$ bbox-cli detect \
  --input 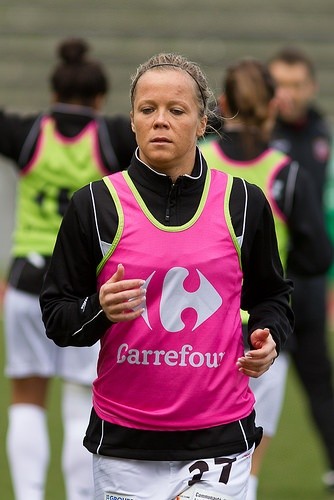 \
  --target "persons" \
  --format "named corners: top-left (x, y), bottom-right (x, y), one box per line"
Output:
top-left (0, 37), bottom-right (224, 500)
top-left (266, 48), bottom-right (333, 488)
top-left (194, 57), bottom-right (333, 499)
top-left (38, 54), bottom-right (298, 500)
